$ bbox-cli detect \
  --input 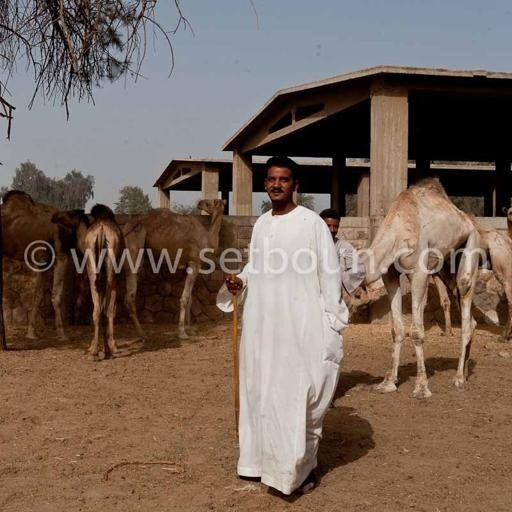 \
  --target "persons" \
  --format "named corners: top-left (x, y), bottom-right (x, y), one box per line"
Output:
top-left (319, 209), bottom-right (367, 302)
top-left (225, 156), bottom-right (349, 495)
